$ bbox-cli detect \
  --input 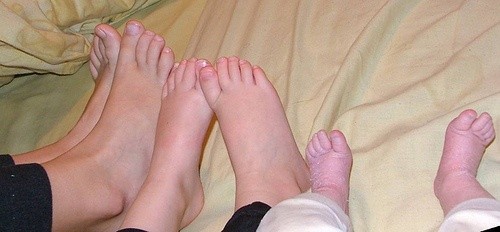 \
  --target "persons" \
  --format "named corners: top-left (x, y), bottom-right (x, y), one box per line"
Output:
top-left (116, 56), bottom-right (313, 232)
top-left (0, 20), bottom-right (175, 232)
top-left (256, 109), bottom-right (500, 232)
